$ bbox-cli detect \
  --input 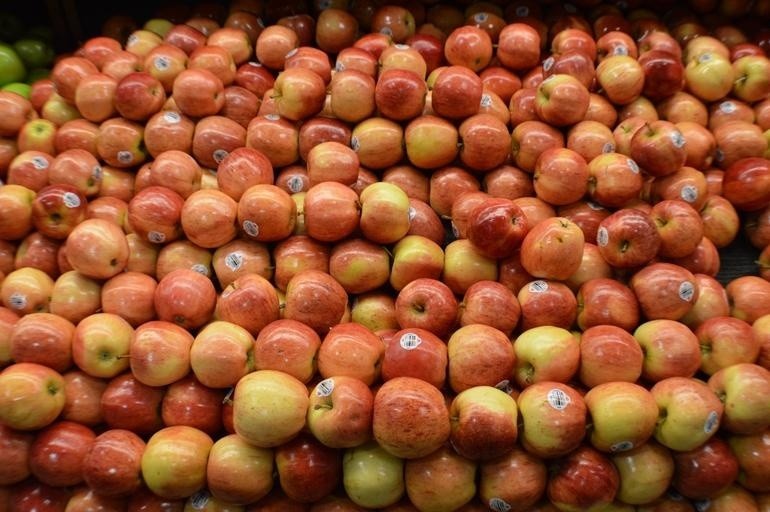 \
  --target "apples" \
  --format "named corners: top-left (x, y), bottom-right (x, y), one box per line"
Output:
top-left (0, 0), bottom-right (770, 512)
